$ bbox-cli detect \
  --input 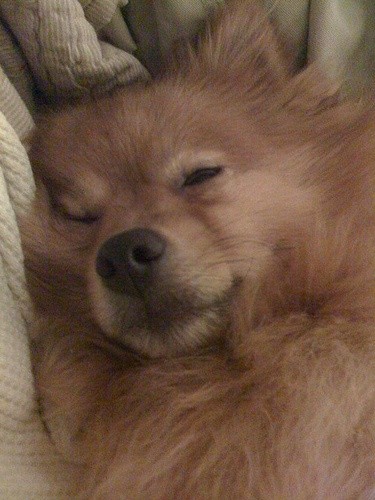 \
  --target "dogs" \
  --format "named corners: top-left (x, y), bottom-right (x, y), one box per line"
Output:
top-left (18, 1), bottom-right (375, 500)
